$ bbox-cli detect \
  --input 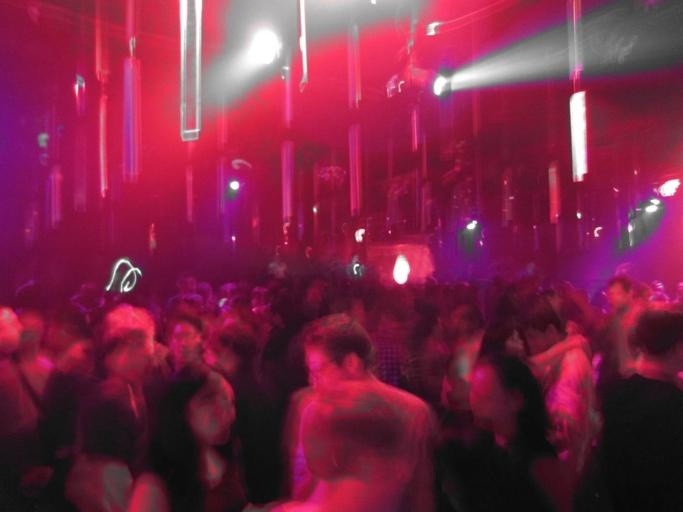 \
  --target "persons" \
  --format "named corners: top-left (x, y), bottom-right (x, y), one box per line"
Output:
top-left (0, 241), bottom-right (682, 511)
